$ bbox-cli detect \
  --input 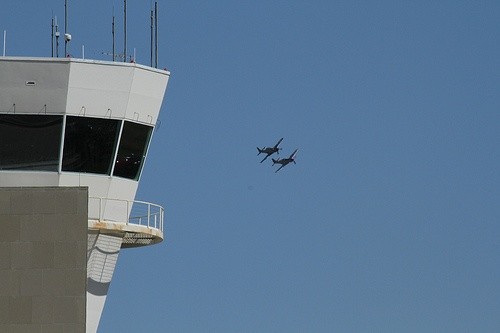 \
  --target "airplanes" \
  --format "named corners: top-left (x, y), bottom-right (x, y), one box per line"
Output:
top-left (272, 149), bottom-right (298, 173)
top-left (256, 138), bottom-right (284, 164)
